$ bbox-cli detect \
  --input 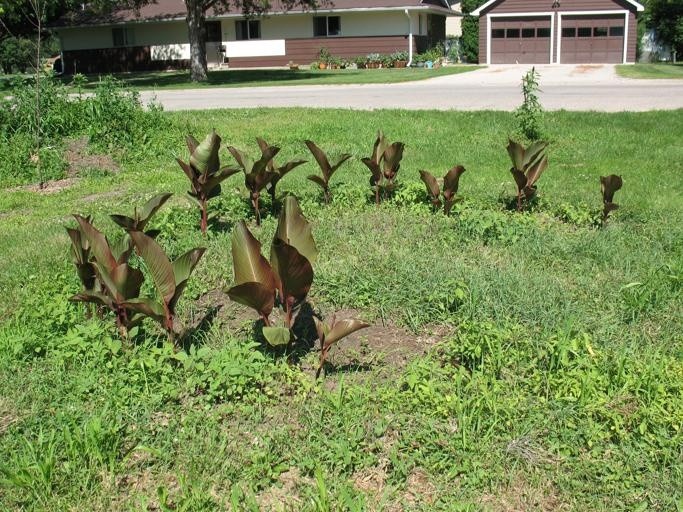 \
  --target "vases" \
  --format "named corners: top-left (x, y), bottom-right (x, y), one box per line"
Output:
top-left (307, 41), bottom-right (472, 70)
